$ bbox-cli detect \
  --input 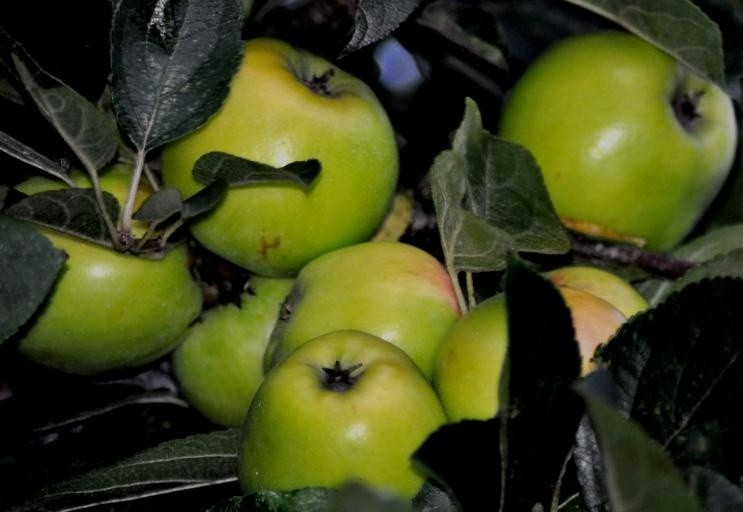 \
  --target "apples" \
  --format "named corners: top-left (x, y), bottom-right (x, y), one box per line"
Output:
top-left (541, 266), bottom-right (649, 320)
top-left (239, 328), bottom-right (448, 501)
top-left (161, 38), bottom-right (399, 280)
top-left (432, 285), bottom-right (627, 423)
top-left (277, 241), bottom-right (462, 385)
top-left (14, 165), bottom-right (203, 373)
top-left (499, 30), bottom-right (736, 256)
top-left (172, 276), bottom-right (294, 427)
